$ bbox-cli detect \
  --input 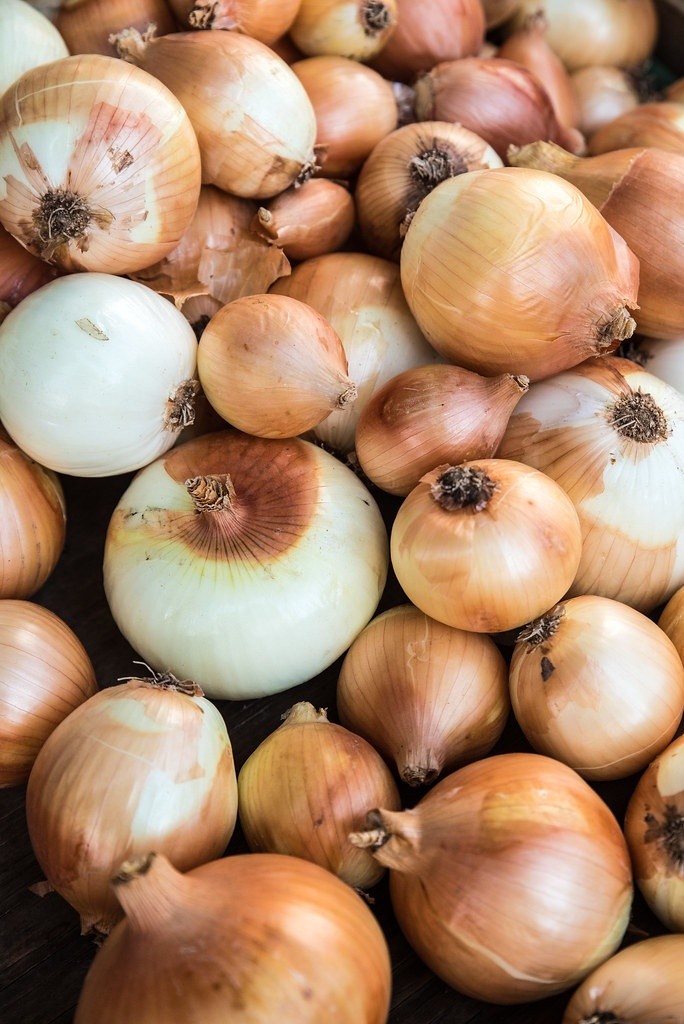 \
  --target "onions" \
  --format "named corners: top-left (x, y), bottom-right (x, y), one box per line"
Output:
top-left (0, 0), bottom-right (684, 1024)
